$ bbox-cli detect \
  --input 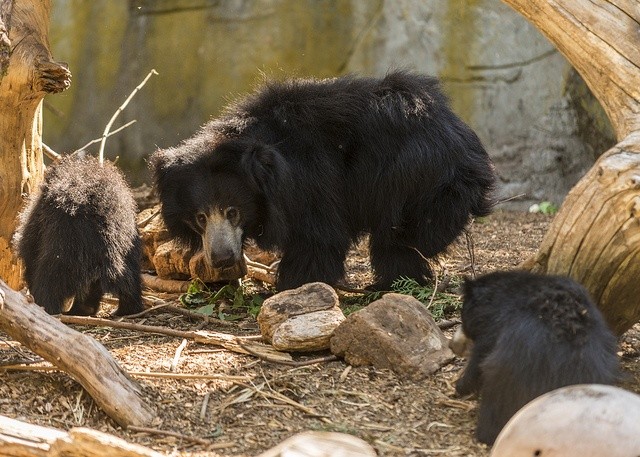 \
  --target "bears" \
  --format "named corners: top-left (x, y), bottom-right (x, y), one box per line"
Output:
top-left (9, 151), bottom-right (145, 326)
top-left (150, 69), bottom-right (496, 298)
top-left (455, 270), bottom-right (624, 446)
